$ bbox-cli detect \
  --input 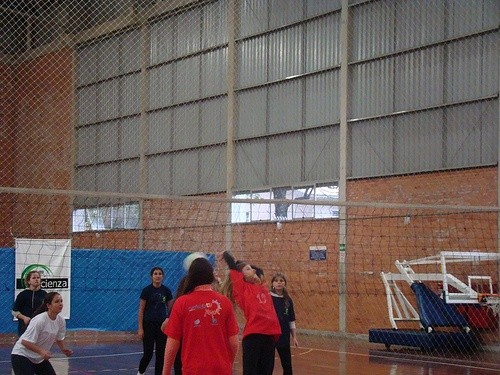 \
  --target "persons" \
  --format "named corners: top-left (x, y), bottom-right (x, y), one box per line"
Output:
top-left (267, 273), bottom-right (299, 375)
top-left (169, 274), bottom-right (193, 375)
top-left (10, 292), bottom-right (73, 375)
top-left (216, 250), bottom-right (282, 375)
top-left (11, 272), bottom-right (49, 338)
top-left (137, 266), bottom-right (174, 375)
top-left (161, 256), bottom-right (239, 375)
